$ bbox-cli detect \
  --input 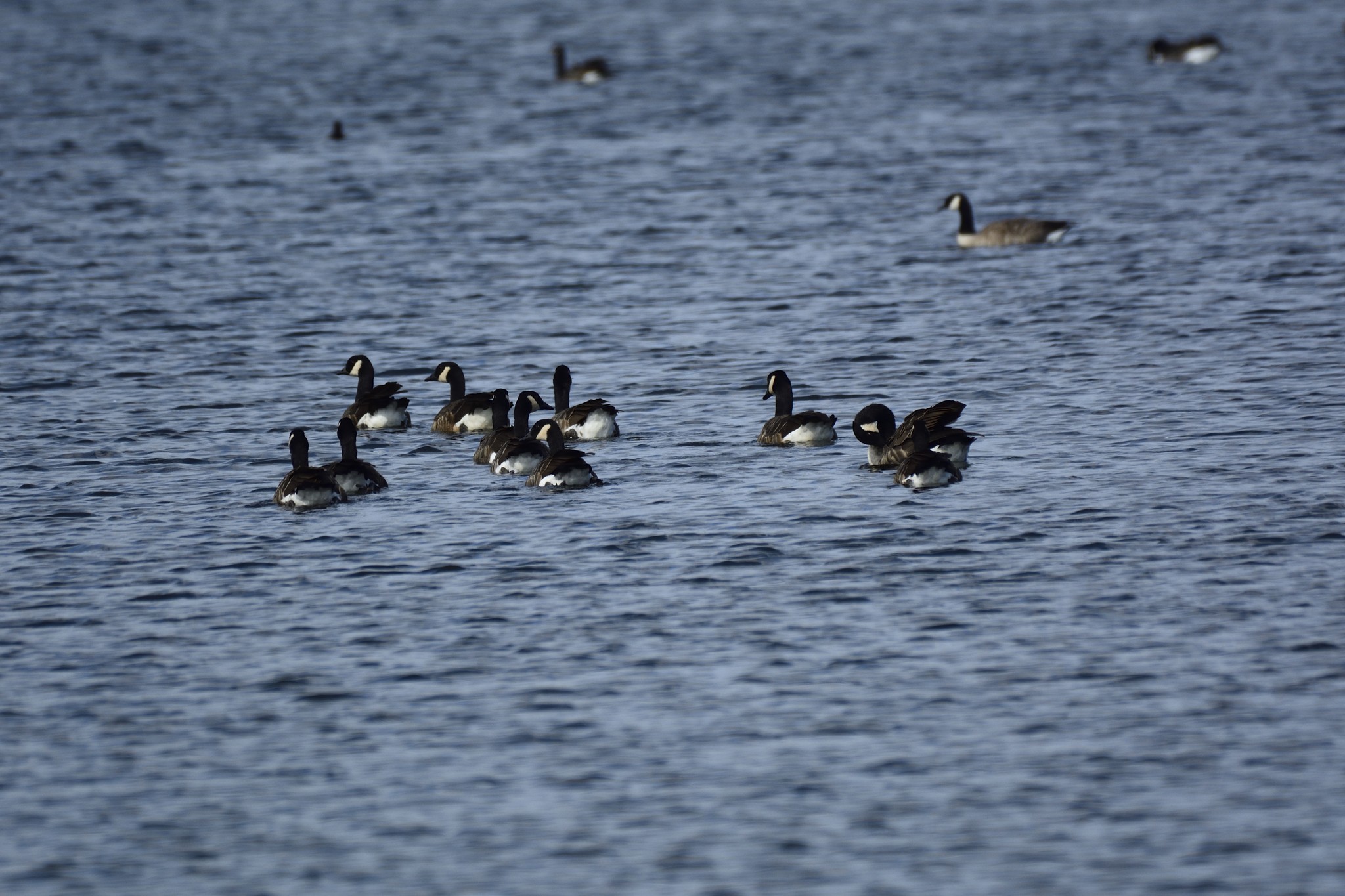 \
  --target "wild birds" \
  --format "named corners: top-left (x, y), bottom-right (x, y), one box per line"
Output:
top-left (336, 354), bottom-right (412, 432)
top-left (424, 362), bottom-right (513, 435)
top-left (473, 390), bottom-right (602, 487)
top-left (757, 370), bottom-right (838, 447)
top-left (536, 365), bottom-right (621, 442)
top-left (272, 417), bottom-right (387, 508)
top-left (936, 193), bottom-right (1081, 248)
top-left (1142, 33), bottom-right (1228, 67)
top-left (853, 399), bottom-right (987, 489)
top-left (326, 41), bottom-right (618, 140)
